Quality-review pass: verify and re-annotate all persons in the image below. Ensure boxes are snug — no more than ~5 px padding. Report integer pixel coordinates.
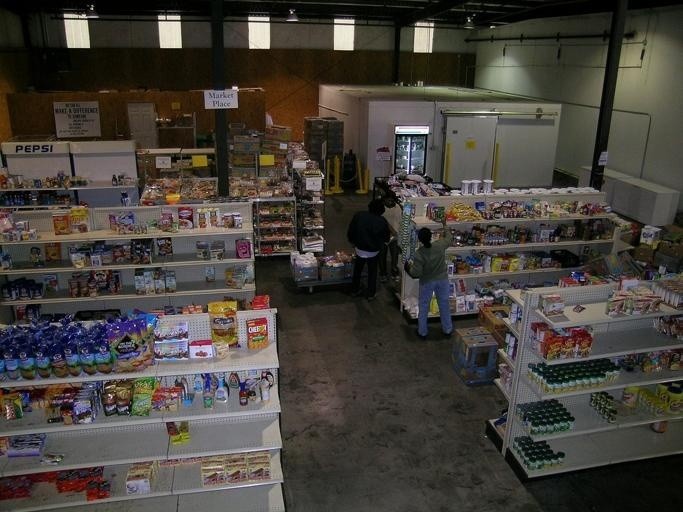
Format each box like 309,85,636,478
408,217,454,339
378,190,403,282
347,198,390,301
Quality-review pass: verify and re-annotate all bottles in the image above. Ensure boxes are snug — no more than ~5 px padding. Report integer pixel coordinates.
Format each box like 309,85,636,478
512,359,621,474
513,225,520,243
203,371,274,410
112,175,117,186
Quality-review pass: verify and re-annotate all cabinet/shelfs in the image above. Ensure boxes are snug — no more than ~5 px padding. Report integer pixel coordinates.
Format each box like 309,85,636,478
578,166,680,227
1,168,220,205
0,307,286,510
484,271,683,478
399,187,622,322
0,200,256,325
228,141,327,255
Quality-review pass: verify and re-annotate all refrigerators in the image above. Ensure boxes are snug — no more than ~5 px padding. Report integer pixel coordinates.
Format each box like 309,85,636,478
395,125,428,175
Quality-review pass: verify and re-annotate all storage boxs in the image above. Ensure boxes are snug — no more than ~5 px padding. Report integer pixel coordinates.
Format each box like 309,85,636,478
231,124,292,150
305,117,344,182
289,262,354,281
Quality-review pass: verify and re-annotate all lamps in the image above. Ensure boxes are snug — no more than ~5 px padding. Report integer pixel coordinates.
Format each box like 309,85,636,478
463,17,475,29
85,5,99,18
286,9,299,22
